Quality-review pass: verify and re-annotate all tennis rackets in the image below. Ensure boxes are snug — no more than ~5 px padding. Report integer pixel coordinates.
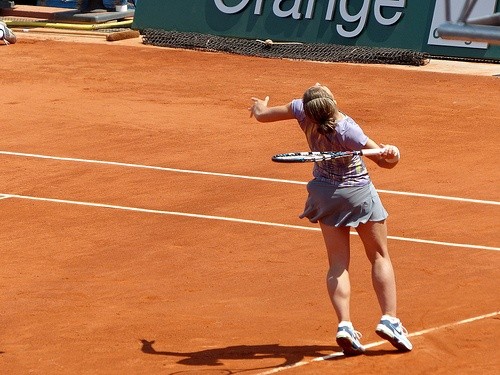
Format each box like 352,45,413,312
271,147,386,164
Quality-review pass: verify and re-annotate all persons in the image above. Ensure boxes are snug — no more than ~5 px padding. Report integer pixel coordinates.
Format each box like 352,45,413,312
248,82,412,353
0,22,17,45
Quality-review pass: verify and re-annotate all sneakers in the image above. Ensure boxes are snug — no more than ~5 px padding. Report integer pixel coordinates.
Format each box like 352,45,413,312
336,327,366,355
375,320,413,351
0,21,17,46
